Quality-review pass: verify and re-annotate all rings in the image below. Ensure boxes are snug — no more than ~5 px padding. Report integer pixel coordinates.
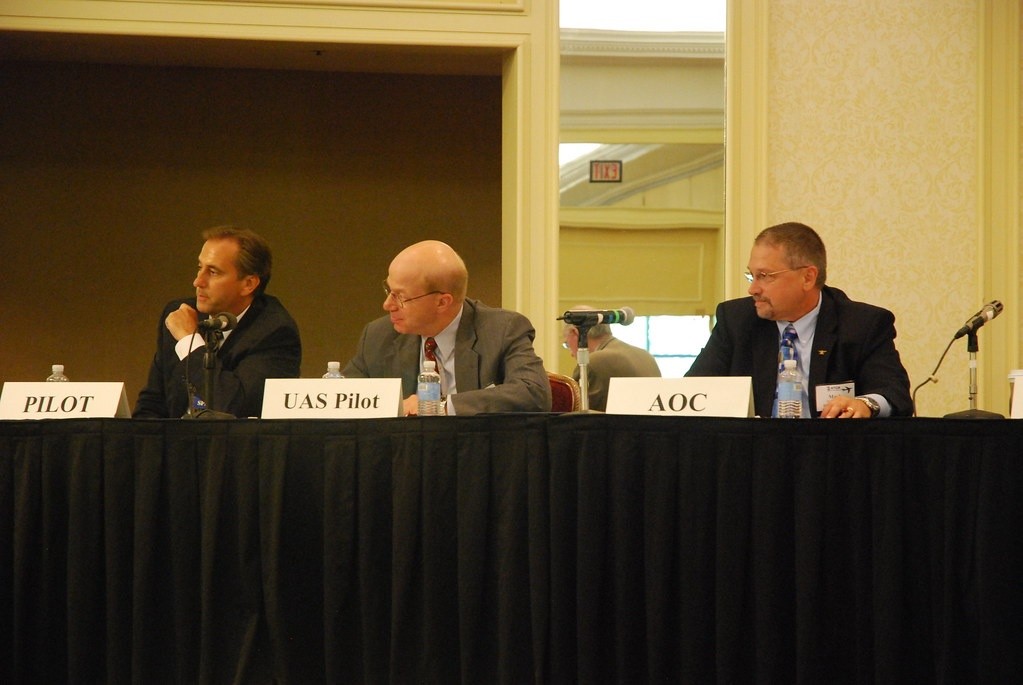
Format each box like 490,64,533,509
847,407,854,412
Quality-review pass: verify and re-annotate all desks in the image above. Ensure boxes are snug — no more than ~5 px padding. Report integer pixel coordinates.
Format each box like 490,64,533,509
0,409,1023,685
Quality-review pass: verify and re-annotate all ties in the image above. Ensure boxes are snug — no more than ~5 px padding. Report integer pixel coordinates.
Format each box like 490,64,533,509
424,337,440,374
779,324,813,418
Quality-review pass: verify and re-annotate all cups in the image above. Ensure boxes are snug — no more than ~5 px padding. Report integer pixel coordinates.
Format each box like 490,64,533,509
1008,368,1023,420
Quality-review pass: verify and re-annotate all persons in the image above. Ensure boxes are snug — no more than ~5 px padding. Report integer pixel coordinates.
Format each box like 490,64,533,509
562,305,661,412
132,225,301,418
341,240,552,416
684,222,915,418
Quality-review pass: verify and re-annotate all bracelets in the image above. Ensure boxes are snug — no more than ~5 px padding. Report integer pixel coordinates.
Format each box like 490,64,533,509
439,396,447,415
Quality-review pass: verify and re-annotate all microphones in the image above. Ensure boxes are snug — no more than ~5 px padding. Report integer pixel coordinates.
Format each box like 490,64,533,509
557,306,635,326
196,312,238,331
955,299,1004,339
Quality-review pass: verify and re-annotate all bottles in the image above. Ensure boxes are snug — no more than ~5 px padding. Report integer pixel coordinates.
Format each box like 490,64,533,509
46,364,70,382
417,361,441,417
779,360,802,419
321,361,344,379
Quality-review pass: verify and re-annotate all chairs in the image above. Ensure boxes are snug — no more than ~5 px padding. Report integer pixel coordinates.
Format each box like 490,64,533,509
546,369,582,411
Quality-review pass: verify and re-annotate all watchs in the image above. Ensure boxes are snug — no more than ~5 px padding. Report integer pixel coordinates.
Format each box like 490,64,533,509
856,397,880,417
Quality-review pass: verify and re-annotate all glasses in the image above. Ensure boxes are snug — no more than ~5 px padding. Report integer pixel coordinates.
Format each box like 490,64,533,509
382,280,445,308
744,265,807,285
563,336,569,349
